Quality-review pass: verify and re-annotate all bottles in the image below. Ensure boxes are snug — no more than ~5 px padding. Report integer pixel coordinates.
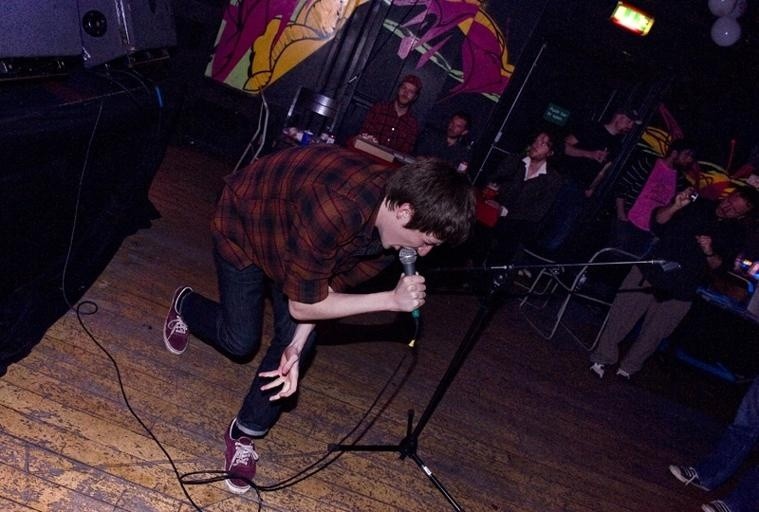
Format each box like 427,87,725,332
455,158,470,173
320,129,337,144
573,270,589,292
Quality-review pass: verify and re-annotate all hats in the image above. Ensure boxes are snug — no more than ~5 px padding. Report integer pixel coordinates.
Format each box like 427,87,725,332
617,106,644,120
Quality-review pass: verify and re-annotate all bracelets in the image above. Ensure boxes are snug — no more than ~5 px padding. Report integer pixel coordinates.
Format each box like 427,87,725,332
703,245,720,258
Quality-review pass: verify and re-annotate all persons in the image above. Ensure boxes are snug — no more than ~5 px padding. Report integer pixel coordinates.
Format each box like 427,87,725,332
542,102,645,260
602,131,701,257
360,72,424,165
665,374,758,511
485,124,565,266
414,110,481,178
587,183,758,382
159,139,479,497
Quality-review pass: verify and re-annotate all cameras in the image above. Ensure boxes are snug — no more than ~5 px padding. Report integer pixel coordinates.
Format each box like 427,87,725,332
690,193,700,203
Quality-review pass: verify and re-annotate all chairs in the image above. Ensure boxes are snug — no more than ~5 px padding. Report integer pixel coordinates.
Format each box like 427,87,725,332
518,246,641,352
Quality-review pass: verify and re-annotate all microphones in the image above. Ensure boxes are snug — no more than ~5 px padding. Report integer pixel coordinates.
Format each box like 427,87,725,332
400,247,419,318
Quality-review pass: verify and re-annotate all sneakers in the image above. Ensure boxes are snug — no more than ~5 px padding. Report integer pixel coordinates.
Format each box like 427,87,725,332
590,361,605,379
616,368,631,380
668,465,710,492
162,284,193,355
702,497,732,512
225,417,259,495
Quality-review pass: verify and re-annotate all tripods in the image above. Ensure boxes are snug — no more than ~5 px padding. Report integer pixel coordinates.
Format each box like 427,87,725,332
328,258,633,512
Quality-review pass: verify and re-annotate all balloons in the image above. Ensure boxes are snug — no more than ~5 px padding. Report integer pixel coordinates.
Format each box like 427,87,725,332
708,0,749,47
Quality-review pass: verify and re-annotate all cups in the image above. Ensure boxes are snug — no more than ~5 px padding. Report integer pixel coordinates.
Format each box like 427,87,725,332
302,130,314,145
483,182,500,200
